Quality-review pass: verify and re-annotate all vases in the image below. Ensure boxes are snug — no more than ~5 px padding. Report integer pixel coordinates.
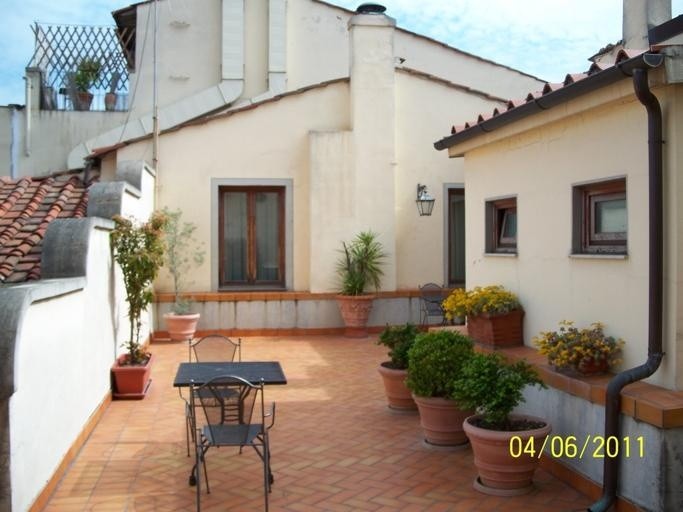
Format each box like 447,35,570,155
465,307,524,347
577,352,609,374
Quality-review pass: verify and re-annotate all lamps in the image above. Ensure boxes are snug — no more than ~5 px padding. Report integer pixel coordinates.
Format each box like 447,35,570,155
415,183,436,216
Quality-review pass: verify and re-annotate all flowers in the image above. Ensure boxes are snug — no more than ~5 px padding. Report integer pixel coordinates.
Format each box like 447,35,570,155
531,320,625,372
440,284,520,321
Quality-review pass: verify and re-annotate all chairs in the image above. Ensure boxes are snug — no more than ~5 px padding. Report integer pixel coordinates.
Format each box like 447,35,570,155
178,334,252,457
418,283,448,330
187,375,275,512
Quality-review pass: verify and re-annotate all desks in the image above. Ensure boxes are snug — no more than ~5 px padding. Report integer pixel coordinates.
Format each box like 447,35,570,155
173,362,287,485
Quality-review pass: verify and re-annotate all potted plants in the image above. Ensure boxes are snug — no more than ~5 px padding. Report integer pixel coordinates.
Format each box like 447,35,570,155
111,210,168,400
373,321,419,413
72,60,101,111
403,327,475,452
104,73,120,111
453,351,552,497
326,228,392,338
162,206,206,342
